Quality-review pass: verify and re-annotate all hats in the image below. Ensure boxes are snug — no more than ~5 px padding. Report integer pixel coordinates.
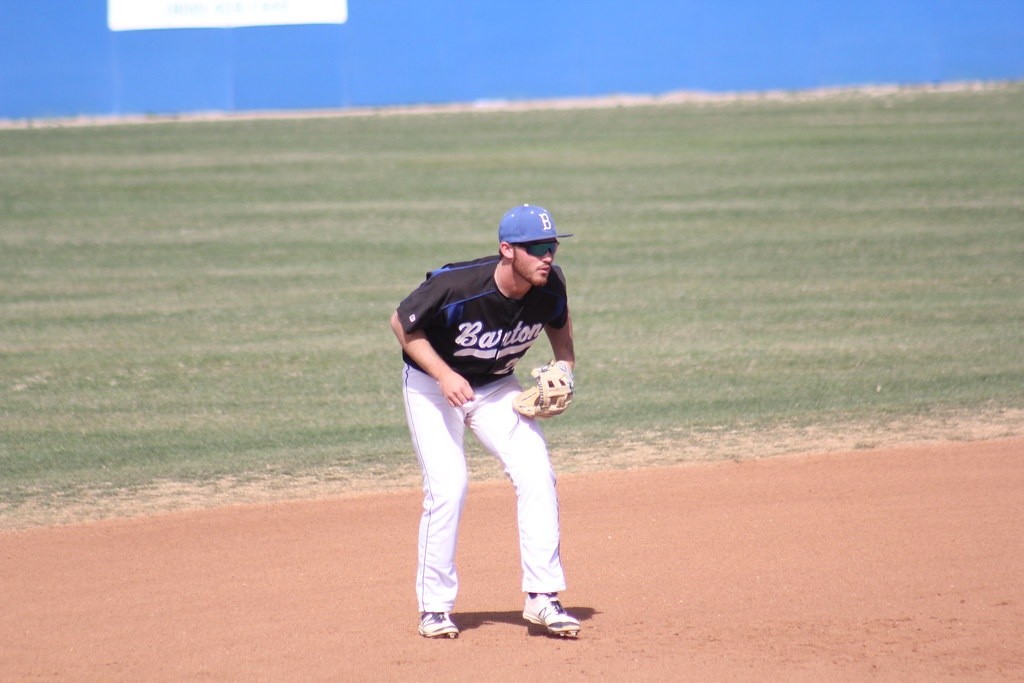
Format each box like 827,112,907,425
498,204,573,244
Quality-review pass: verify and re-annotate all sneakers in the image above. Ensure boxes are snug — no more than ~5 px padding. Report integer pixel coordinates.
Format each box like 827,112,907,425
522,593,581,637
417,611,459,639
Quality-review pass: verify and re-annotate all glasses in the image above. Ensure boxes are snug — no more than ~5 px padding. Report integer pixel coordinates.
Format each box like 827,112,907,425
514,240,559,257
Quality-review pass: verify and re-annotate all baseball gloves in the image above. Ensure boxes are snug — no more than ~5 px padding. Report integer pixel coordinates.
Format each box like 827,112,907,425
512,360,575,420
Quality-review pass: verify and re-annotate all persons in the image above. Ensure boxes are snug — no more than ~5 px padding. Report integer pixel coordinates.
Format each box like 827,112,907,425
390,203,582,640
